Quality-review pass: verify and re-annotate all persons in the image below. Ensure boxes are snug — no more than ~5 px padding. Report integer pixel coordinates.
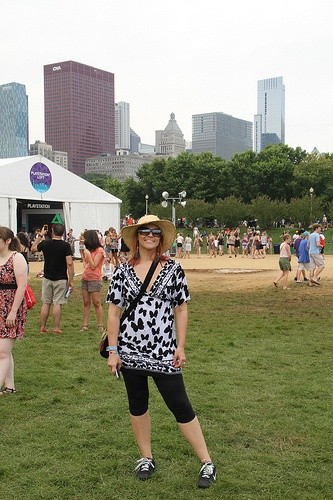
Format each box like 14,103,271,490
105,214,216,487
0,214,329,394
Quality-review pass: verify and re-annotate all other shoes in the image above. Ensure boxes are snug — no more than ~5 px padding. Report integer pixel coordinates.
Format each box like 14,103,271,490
0,387,16,395
98,325,104,332
79,326,88,331
53,329,63,333
310,278,321,285
39,328,48,333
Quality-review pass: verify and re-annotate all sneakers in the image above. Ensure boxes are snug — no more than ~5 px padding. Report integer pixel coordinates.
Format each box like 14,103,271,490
133,456,155,480
197,458,216,488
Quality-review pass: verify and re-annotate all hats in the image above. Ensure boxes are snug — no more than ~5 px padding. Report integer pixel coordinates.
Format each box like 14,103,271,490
121,215,176,253
302,231,309,238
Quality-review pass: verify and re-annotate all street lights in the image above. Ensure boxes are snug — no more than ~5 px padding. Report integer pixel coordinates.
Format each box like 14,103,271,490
161,190,186,228
309,187,314,224
145,195,149,215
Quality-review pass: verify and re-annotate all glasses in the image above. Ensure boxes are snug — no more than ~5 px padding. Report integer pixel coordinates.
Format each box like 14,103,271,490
137,227,162,237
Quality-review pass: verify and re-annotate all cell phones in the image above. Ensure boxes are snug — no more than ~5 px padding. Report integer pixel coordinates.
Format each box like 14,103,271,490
115,366,120,379
44,224,48,234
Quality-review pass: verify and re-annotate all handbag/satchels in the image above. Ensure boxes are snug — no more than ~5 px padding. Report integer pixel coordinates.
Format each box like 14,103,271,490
99,327,113,358
25,285,36,309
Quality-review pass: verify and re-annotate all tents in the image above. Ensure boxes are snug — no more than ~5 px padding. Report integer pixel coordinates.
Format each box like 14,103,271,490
0,155,123,260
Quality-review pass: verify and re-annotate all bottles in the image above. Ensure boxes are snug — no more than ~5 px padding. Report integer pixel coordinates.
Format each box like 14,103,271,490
65,286,73,298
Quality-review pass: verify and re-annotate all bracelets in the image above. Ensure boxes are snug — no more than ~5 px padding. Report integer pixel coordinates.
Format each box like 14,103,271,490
105,346,118,356
38,234,43,238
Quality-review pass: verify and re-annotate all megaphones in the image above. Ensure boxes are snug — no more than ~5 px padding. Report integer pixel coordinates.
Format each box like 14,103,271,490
161,200,168,208
161,191,169,199
178,200,186,208
178,190,187,198
